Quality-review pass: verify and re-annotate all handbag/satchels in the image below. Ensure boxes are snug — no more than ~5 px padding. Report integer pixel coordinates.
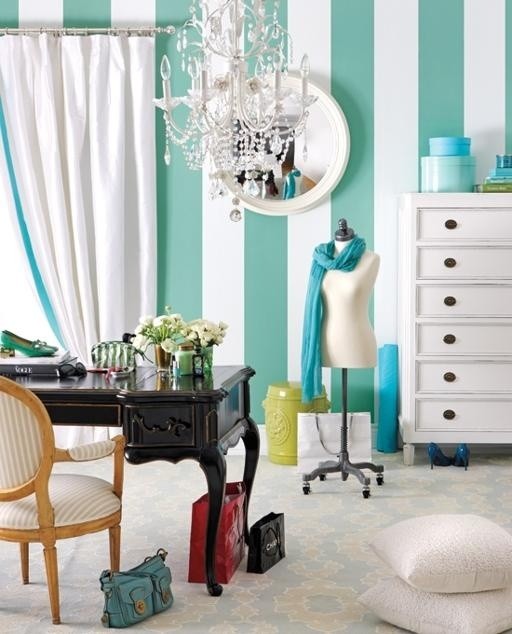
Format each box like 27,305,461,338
100,548,173,628
188,481,246,583
247,512,285,573
92,340,136,369
297,412,372,473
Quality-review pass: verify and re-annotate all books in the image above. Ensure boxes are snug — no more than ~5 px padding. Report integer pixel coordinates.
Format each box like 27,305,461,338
474,177,512,193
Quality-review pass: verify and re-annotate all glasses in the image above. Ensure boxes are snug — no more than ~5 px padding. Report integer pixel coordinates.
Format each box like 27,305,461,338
59,363,87,378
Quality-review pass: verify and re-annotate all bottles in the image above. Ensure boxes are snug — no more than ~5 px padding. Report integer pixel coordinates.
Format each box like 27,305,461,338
192,347,203,379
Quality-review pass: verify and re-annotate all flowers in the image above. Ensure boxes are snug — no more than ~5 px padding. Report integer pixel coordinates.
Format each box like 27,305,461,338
170,316,231,349
129,305,188,354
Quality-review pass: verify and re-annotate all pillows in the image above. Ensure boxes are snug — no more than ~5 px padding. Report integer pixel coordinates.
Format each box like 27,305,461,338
355,575,512,634
363,511,512,596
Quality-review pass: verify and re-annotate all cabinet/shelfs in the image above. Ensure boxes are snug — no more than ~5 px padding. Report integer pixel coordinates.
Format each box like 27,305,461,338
393,190,512,469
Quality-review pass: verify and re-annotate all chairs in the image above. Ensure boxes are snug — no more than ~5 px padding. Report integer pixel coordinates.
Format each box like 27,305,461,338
0,375,129,627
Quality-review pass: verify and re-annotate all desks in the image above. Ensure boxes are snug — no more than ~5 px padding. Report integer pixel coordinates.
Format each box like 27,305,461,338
8,363,262,599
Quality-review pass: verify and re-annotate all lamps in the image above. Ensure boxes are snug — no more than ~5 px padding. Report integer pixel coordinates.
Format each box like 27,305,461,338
158,1,322,225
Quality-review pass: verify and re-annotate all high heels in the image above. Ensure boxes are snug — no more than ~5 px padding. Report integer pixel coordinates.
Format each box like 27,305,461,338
1,330,58,357
427,443,453,469
453,444,470,470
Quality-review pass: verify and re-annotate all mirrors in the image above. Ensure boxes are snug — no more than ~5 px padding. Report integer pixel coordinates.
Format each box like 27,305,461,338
209,71,354,218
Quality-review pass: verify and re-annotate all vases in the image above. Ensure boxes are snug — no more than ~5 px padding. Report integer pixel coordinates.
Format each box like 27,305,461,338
202,346,215,371
153,345,174,373
